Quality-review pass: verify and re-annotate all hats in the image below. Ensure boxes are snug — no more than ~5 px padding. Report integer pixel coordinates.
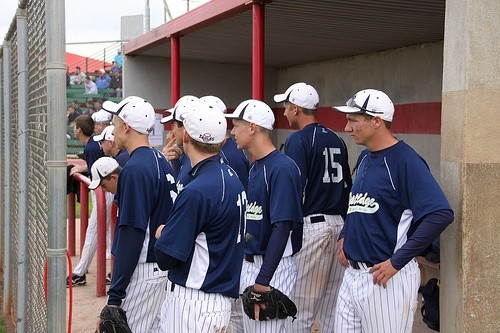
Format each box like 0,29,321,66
166,94,199,113
201,96,227,113
88,157,119,189
94,108,111,123
93,125,115,142
273,82,319,110
180,106,227,144
332,88,395,123
160,104,192,123
224,99,275,131
102,96,155,135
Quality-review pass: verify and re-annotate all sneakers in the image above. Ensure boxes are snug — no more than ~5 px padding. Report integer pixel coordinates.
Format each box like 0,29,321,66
66,273,87,287
104,274,112,284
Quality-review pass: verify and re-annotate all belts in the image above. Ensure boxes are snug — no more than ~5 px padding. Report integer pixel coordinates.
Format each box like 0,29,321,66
311,216,325,223
244,254,265,263
348,259,376,269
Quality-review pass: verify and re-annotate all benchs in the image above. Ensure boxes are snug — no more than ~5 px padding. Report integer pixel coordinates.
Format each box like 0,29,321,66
67,67,122,158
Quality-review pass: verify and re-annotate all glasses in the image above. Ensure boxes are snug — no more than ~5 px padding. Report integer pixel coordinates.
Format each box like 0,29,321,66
346,97,382,114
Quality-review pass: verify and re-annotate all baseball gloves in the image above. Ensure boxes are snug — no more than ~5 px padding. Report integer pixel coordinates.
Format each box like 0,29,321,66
241,285,298,322
95,305,132,333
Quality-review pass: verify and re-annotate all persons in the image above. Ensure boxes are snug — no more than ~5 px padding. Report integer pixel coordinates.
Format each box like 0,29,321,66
223,100,304,333
274,83,352,333
66,96,250,333
66,49,122,99
333,88,454,333
